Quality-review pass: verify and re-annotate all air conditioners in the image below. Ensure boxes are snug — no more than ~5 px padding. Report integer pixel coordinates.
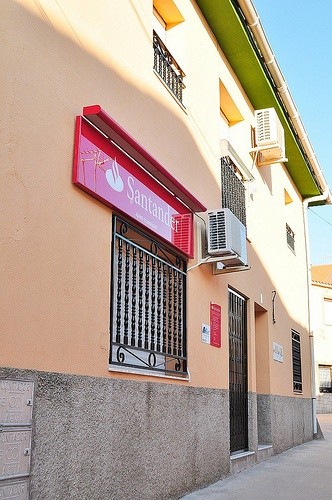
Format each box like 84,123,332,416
254,109,286,162
207,210,250,267
